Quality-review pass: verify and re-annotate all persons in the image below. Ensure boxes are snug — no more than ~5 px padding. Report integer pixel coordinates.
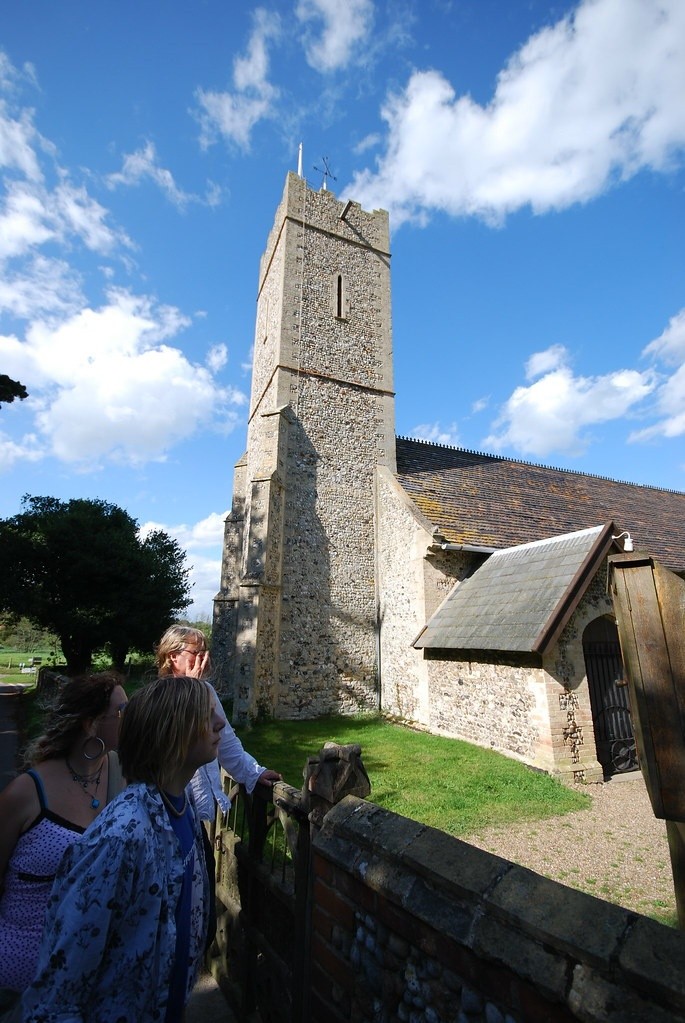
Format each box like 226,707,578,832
23,674,230,1023
145,621,282,952
0,674,130,1006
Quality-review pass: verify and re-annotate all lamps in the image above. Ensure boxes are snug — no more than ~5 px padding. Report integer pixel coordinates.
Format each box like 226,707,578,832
612,530,633,551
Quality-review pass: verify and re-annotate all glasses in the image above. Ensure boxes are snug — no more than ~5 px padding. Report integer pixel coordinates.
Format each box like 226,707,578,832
183,649,205,657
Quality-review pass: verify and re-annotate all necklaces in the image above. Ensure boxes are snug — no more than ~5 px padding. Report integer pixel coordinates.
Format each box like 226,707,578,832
64,754,105,809
156,781,188,816
